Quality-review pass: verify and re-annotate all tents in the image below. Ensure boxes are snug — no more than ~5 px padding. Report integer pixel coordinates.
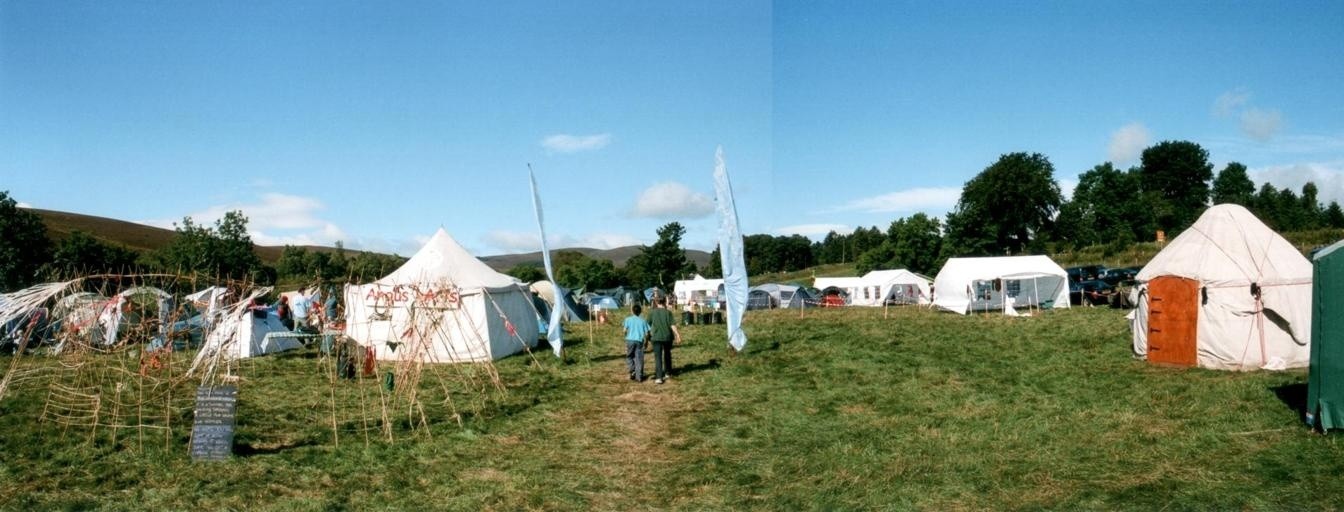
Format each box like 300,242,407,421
340,224,541,365
528,274,860,335
0,284,323,363
849,268,933,307
1126,201,1314,373
1302,237,1344,434
931,253,1071,316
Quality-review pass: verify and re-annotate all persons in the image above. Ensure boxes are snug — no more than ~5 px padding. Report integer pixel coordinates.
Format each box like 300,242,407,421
325,284,342,329
646,293,682,385
621,304,651,382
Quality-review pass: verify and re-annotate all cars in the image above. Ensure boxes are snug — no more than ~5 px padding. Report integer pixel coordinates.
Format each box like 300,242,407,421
1065,263,1143,308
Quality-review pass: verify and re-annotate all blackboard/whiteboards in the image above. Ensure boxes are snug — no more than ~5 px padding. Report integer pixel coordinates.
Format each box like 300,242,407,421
186,386,238,463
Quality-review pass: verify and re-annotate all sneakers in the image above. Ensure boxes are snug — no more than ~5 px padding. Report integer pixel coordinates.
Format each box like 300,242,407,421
624,371,674,385
